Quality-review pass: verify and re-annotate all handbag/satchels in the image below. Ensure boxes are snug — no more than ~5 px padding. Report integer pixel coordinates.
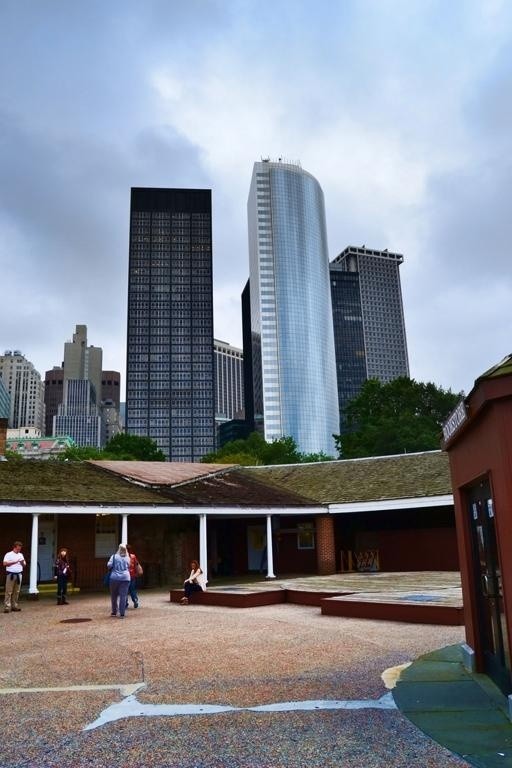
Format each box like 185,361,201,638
134,560,143,577
103,567,113,585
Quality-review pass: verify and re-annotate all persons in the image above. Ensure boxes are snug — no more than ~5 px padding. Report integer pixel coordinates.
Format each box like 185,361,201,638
126,544,139,608
107,543,131,618
54,547,70,605
3,540,26,613
180,560,206,605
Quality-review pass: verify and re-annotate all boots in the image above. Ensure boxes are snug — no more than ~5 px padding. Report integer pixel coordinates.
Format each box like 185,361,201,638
57,596,68,605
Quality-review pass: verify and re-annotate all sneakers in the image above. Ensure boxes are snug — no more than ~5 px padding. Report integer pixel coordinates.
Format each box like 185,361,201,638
180,597,188,604
4,608,21,613
111,601,138,618
